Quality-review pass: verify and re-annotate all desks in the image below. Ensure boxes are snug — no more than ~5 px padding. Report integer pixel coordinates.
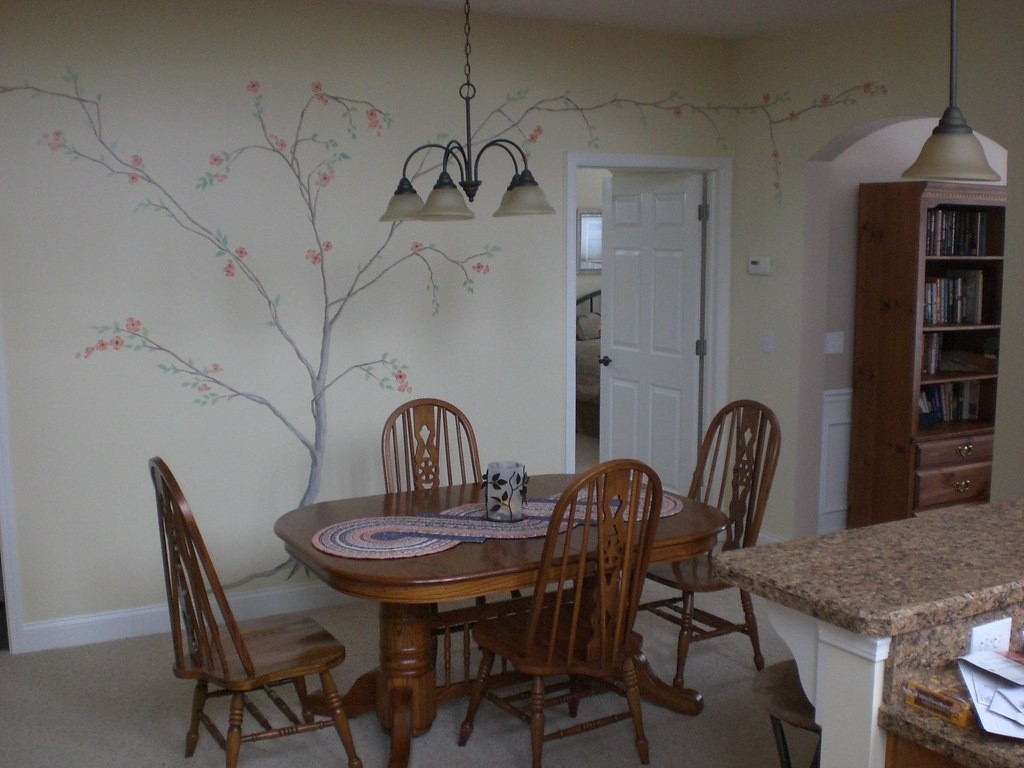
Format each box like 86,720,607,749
274,473,729,768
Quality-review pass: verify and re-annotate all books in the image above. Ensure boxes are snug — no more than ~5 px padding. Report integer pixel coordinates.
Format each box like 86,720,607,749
921,332,944,374
927,209,988,256
920,382,980,423
924,270,983,327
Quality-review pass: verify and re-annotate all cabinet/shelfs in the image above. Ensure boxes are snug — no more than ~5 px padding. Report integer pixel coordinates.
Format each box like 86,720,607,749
844,182,1008,530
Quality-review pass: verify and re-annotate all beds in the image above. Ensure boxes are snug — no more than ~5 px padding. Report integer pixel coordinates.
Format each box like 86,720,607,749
576,290,601,438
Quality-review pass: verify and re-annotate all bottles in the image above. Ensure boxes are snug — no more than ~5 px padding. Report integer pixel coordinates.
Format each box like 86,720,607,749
481,461,530,522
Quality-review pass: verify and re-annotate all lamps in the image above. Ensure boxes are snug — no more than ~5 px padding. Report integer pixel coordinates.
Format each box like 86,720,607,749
378,0,559,221
901,0,1001,181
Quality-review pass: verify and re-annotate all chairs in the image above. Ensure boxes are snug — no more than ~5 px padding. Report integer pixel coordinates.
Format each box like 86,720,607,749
642,399,781,690
381,399,524,653
148,457,364,768
455,458,662,768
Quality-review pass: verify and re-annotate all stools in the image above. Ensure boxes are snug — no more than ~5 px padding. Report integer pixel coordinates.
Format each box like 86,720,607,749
753,658,822,768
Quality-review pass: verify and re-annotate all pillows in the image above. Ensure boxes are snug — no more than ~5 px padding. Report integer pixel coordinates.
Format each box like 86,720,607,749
576,312,601,341
